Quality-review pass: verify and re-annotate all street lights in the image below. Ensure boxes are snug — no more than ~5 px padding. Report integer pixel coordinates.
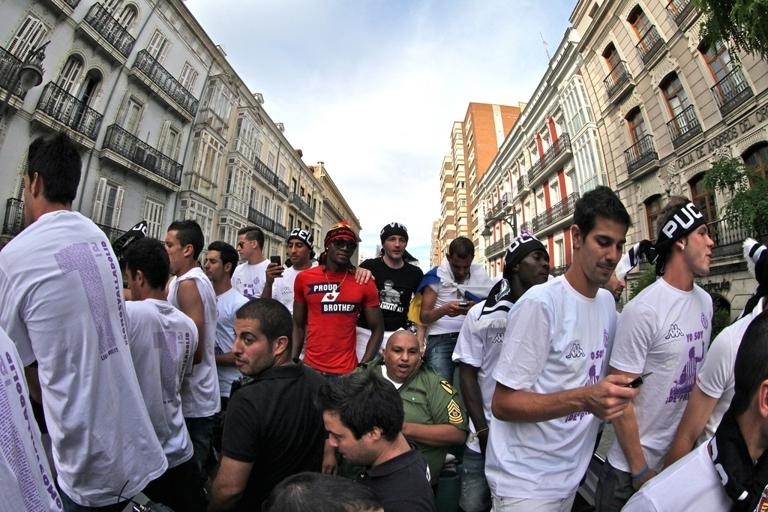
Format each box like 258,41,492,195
1,36,53,122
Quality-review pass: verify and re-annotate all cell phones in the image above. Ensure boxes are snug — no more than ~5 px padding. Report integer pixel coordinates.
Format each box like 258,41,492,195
459,302,468,307
270,256,282,275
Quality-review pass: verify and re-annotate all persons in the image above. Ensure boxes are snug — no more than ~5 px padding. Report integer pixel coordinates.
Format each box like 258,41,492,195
669,341,704,402
663,238,768,469
594,196,714,512
0,132,170,512
585,360,604,385
484,186,640,512
203,299,470,512
0,327,64,512
604,271,627,303
124,236,200,504
123,220,222,465
204,221,556,484
621,309,768,512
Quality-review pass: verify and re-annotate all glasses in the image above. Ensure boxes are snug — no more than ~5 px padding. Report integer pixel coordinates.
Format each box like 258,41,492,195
330,238,357,251
239,239,252,249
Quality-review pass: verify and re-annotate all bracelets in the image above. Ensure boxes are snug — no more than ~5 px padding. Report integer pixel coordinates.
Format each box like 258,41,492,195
631,465,649,480
